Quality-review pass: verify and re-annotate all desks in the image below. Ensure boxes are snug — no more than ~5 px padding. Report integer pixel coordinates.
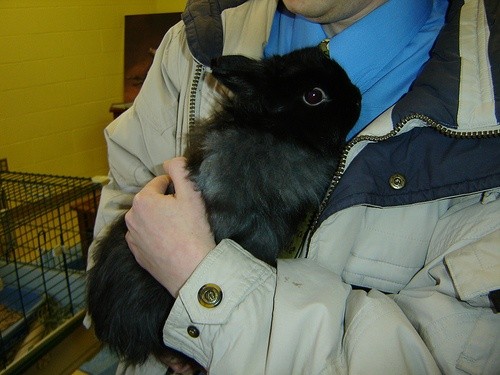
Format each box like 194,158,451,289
110,102,133,120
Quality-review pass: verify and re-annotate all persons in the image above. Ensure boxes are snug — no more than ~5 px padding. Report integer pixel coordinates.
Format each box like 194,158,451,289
83,0,500,374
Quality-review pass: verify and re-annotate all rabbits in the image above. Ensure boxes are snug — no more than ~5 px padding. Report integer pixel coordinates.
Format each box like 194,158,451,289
86,43,362,366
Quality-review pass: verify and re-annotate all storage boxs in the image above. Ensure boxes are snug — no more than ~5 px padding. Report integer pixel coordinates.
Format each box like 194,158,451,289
70,193,100,258
